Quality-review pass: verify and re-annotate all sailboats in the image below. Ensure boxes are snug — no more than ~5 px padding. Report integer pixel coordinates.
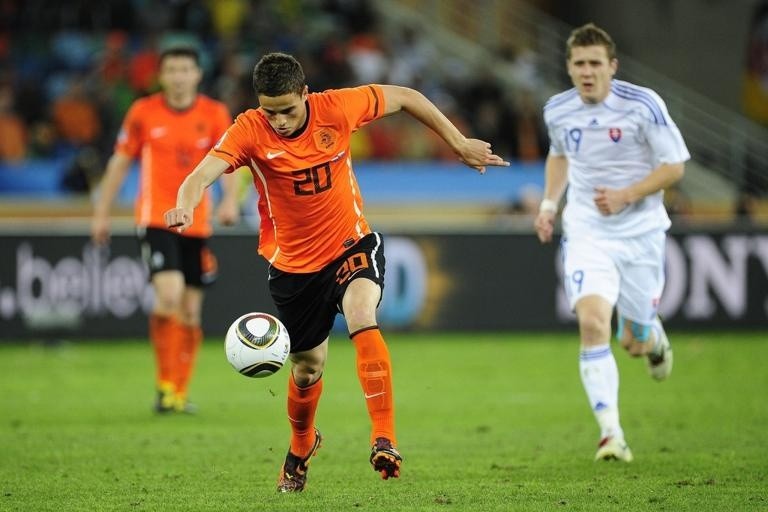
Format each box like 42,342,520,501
225,312,290,378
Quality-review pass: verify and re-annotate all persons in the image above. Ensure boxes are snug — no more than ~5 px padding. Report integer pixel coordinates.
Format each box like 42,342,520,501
162,53,512,496
531,21,692,464
90,42,241,416
0,0,766,226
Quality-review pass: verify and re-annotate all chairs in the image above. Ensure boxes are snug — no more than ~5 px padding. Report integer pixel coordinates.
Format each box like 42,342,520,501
593,432,635,467
275,426,322,494
645,311,672,382
369,434,403,482
155,382,187,413
